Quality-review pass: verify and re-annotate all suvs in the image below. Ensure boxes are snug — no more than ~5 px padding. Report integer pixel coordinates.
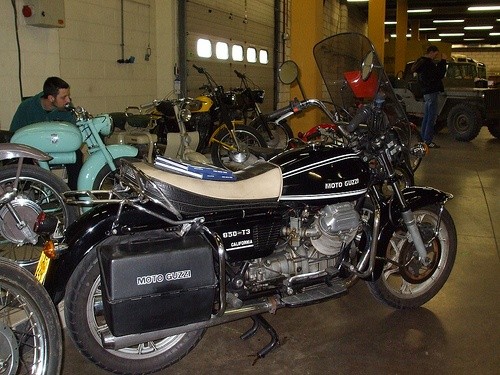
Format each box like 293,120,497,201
391,57,500,142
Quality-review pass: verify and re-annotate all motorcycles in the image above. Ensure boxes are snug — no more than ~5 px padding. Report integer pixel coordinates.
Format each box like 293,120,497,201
9,103,138,214
0,142,81,271
97,96,209,166
150,64,268,172
0,257,65,375
219,70,295,157
62,61,457,375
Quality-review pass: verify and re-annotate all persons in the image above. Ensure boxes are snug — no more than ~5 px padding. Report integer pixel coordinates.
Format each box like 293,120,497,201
7,76,84,191
415,44,448,149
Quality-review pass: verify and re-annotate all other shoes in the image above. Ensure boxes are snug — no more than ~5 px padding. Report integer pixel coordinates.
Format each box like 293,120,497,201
425,141,440,149
